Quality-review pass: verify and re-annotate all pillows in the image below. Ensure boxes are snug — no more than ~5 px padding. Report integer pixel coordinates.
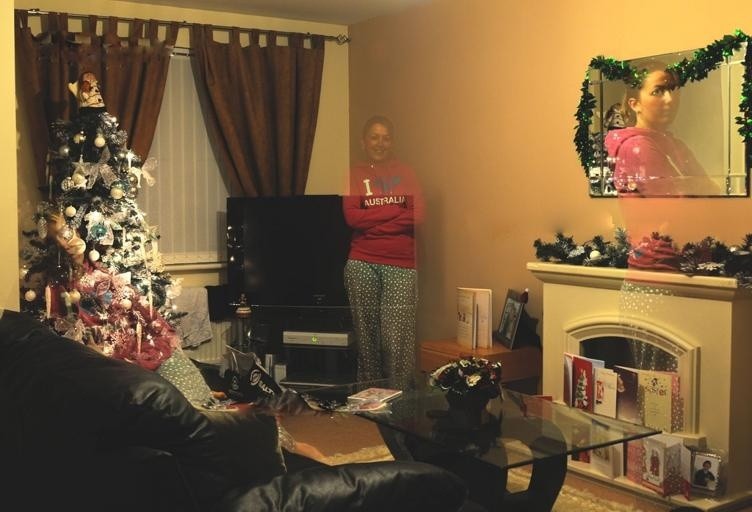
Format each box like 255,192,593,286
155,347,216,412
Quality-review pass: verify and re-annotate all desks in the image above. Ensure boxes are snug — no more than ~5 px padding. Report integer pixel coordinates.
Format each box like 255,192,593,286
419,334,542,396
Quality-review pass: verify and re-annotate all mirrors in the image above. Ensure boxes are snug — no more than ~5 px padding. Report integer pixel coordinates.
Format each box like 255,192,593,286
588,41,751,199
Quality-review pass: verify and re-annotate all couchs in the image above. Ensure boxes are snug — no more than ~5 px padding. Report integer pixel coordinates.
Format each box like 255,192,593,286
0,310,469,511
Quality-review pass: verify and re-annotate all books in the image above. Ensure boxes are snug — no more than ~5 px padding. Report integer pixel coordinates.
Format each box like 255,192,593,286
455,286,494,350
347,386,404,404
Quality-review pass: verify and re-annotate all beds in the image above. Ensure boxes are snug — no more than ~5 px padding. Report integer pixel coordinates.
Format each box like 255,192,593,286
301,369,662,512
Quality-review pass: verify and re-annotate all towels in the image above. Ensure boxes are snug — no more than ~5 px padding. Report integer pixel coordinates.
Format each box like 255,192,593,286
172,287,212,348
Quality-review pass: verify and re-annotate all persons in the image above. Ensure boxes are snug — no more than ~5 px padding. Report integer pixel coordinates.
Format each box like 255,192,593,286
693,460,716,489
500,306,518,339
41,210,255,422
603,61,722,498
342,114,428,422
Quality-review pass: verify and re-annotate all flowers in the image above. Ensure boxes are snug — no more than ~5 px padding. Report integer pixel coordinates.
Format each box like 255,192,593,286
427,355,502,395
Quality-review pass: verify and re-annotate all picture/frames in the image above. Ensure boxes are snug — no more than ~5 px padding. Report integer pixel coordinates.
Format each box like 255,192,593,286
495,289,525,350
691,451,724,492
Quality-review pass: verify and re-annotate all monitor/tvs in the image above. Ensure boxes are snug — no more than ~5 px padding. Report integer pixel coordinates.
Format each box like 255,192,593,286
225,192,420,328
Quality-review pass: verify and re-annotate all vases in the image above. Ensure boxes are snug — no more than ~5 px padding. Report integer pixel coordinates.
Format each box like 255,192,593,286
429,391,503,411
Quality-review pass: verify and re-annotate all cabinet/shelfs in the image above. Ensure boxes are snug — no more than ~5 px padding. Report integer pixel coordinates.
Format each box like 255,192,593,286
231,304,412,402
